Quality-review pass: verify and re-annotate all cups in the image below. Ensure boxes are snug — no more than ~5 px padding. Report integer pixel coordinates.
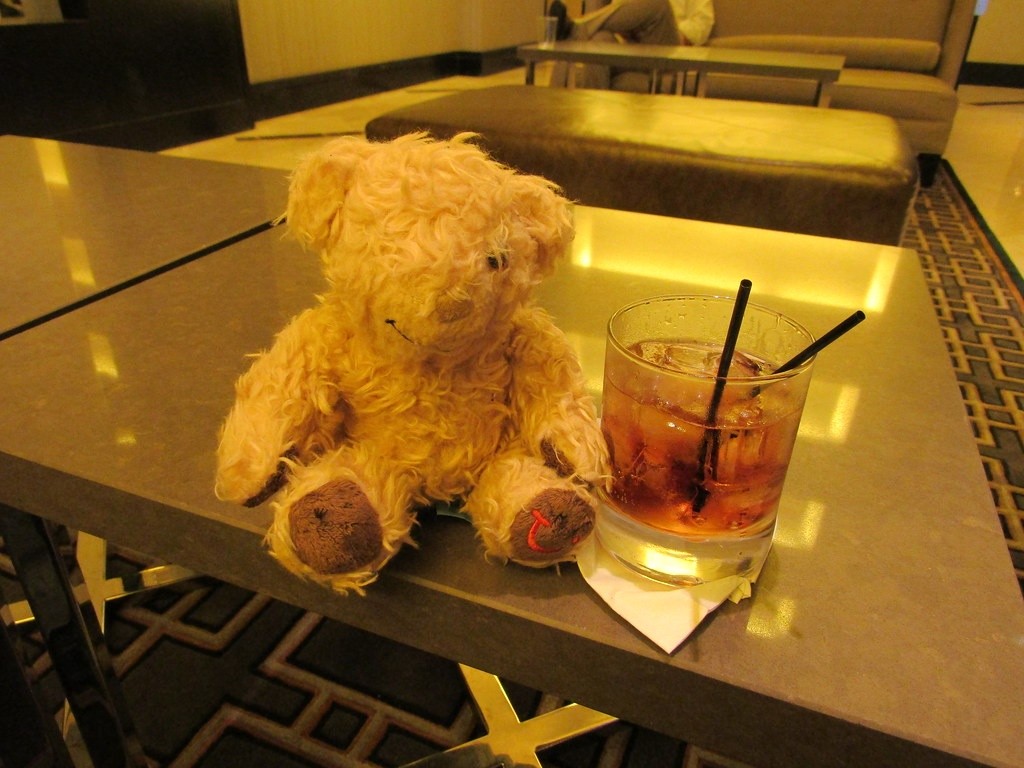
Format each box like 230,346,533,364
596,296,815,586
537,16,558,50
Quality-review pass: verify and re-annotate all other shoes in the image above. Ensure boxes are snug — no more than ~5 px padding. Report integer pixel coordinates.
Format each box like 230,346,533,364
550,0,573,42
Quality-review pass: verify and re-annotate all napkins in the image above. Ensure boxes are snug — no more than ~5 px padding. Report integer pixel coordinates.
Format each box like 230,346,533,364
575,530,771,659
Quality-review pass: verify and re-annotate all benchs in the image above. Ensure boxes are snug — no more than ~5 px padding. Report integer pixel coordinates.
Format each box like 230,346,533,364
544,0,978,188
362,84,920,247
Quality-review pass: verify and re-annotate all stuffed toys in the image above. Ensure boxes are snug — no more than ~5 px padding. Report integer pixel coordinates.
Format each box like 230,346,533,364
214,129,615,596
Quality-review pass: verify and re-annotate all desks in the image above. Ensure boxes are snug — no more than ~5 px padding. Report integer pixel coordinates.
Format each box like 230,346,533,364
519,38,847,109
0,132,1024,768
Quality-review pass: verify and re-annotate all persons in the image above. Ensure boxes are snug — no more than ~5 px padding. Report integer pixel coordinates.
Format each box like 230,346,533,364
542,0,715,94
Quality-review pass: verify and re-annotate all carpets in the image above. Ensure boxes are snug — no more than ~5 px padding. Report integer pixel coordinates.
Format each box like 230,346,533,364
0,159,1024,768
